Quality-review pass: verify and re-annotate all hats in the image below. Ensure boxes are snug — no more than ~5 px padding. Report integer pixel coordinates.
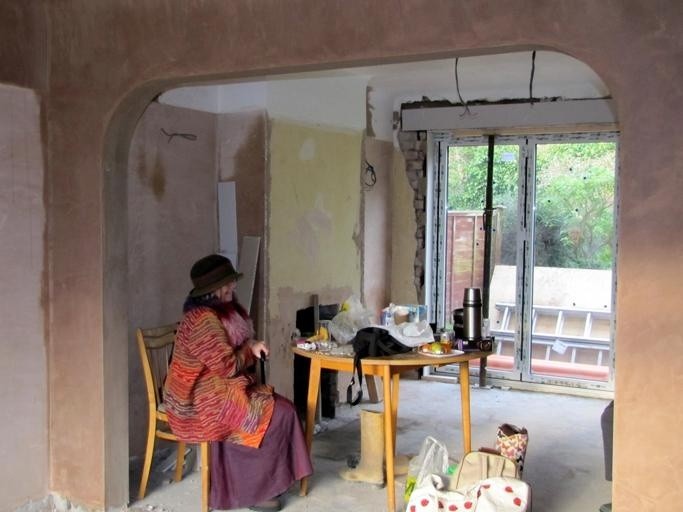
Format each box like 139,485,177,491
187,253,244,296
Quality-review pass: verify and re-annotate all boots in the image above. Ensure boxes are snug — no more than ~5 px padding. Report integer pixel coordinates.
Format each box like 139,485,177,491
337,408,411,490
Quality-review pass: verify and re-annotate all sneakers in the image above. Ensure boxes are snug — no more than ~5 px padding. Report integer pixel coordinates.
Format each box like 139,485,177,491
250,498,281,512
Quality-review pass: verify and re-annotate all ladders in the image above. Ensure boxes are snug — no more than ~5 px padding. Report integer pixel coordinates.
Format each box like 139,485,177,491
491,302,611,368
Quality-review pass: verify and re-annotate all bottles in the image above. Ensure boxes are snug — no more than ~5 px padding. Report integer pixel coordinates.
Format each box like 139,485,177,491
462,287,481,348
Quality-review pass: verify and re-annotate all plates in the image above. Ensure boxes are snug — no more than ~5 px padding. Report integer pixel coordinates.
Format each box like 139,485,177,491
412,347,465,358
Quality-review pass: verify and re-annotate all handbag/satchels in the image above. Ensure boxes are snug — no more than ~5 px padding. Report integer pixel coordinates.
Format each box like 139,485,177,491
450,450,519,493
346,326,413,406
495,423,527,480
329,309,371,343
404,474,530,512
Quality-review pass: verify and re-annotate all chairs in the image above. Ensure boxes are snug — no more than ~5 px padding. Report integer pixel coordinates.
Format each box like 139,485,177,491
136,324,214,511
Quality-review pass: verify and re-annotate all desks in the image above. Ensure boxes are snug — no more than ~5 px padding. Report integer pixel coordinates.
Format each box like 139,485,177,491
288,339,495,511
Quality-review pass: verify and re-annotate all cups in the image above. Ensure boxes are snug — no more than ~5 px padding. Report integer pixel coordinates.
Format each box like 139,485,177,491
314,319,332,353
440,334,452,353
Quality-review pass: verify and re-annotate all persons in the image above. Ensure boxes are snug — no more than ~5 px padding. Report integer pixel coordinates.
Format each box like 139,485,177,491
163,253,314,512
